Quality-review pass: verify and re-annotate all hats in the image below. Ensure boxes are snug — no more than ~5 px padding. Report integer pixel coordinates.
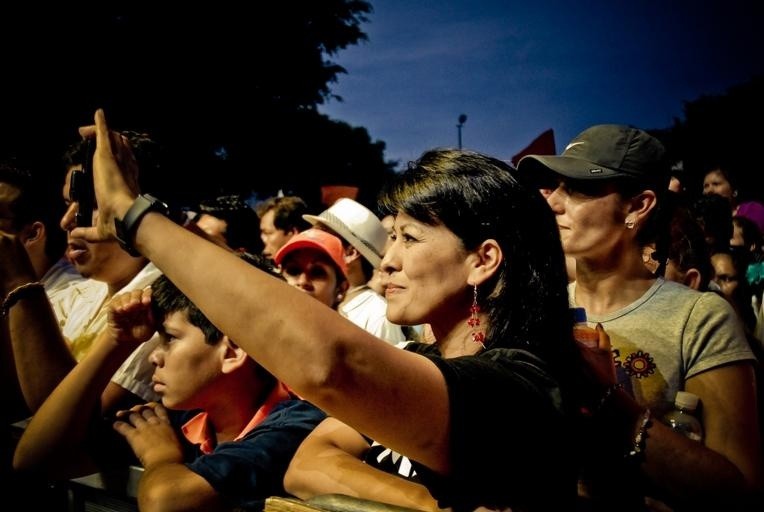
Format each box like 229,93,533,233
732,201,764,234
274,197,388,279
517,124,672,180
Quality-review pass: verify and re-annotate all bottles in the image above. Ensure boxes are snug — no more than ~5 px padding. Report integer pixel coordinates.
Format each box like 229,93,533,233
659,389,705,446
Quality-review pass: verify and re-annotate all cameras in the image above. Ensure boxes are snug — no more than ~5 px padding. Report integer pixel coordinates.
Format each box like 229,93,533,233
67,137,96,227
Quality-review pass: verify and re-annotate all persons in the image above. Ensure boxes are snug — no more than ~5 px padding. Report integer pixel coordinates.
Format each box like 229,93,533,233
1,107,763,511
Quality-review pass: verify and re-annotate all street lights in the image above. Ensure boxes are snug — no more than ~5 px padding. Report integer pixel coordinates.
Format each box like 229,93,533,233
456,114,468,149
